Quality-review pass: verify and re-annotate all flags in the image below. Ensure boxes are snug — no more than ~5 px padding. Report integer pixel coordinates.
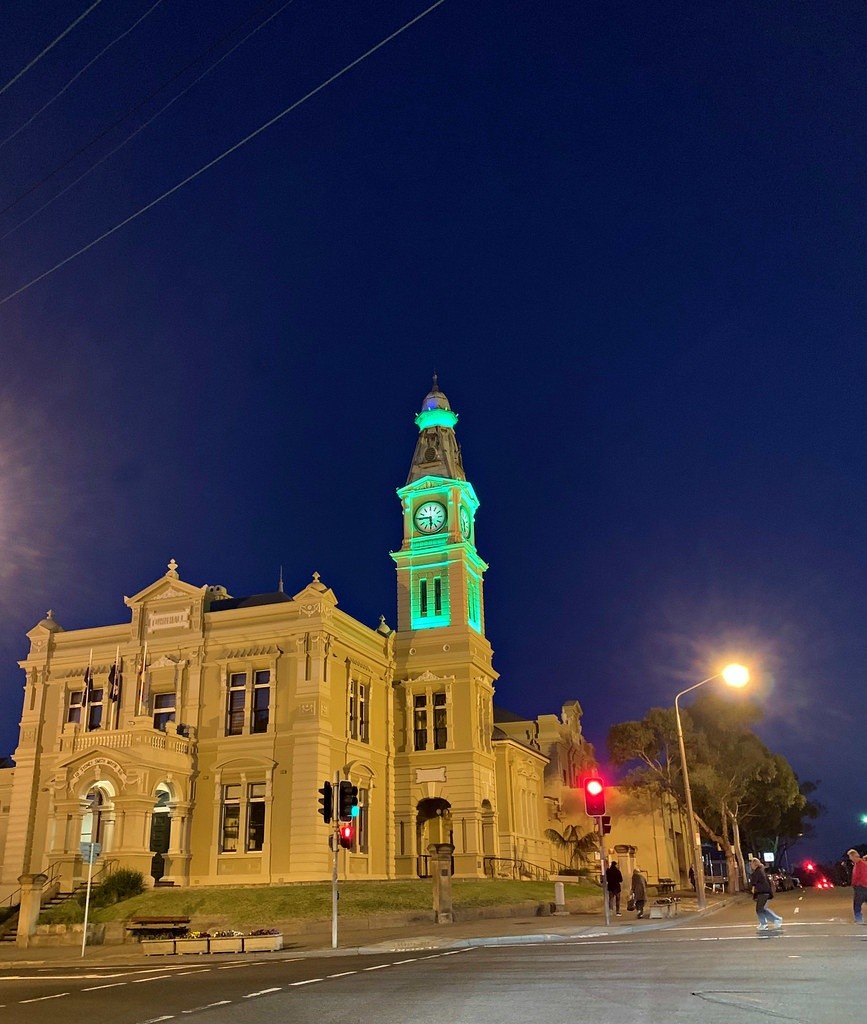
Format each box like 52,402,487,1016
108,654,119,703
138,658,149,702
82,664,94,707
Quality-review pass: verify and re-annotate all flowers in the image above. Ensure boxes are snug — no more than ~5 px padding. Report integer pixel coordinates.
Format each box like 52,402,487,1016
248,928,279,936
177,931,207,939
214,930,244,937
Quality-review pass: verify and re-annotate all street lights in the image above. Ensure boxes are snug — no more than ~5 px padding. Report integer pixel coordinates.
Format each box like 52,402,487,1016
675,663,751,908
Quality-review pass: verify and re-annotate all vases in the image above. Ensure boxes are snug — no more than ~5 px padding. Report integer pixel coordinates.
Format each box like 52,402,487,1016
242,933,283,954
175,937,209,955
209,936,243,954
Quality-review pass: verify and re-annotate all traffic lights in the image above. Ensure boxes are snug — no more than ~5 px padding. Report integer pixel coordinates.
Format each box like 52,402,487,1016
584,776,606,817
600,816,611,836
341,827,353,849
318,781,332,823
339,780,359,823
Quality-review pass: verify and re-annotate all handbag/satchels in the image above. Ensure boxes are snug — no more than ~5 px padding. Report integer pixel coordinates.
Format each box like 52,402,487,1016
627,893,636,911
753,882,774,900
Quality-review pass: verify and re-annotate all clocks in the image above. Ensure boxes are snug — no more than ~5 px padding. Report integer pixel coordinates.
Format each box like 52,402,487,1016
460,505,470,539
413,500,448,535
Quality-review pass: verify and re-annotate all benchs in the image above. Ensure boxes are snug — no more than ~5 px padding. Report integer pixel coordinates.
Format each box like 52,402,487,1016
125,916,188,936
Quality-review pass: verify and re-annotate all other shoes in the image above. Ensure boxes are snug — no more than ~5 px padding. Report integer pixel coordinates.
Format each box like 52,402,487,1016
774,918,782,928
637,909,641,916
616,912,622,916
852,919,865,924
638,915,642,919
756,923,769,930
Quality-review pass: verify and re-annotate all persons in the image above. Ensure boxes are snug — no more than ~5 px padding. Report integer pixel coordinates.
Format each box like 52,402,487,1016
839,849,867,923
605,861,623,916
630,868,647,919
747,858,782,930
689,863,696,892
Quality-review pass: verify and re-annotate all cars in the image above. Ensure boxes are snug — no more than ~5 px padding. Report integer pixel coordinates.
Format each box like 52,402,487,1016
759,866,794,894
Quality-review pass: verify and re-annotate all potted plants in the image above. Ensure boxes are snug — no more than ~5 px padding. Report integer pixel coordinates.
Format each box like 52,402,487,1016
139,934,175,956
